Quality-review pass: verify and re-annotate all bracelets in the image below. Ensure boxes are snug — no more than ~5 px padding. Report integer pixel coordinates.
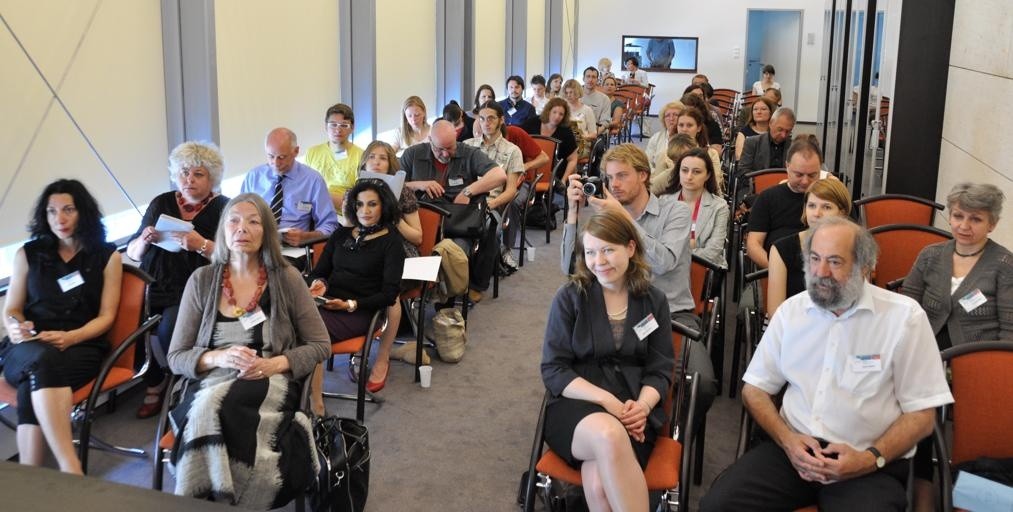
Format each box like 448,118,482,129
199,239,208,254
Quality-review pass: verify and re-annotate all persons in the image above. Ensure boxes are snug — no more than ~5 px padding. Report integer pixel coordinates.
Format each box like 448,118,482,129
646,63,823,269
168,193,332,512
305,178,404,417
561,143,719,444
540,210,676,512
127,141,234,416
247,128,340,274
751,185,848,446
299,58,647,304
899,182,1013,482
646,39,675,69
0,178,121,475
358,143,421,393
696,214,956,512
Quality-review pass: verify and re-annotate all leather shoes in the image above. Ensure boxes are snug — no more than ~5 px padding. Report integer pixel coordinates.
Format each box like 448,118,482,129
136,386,166,418
366,363,390,391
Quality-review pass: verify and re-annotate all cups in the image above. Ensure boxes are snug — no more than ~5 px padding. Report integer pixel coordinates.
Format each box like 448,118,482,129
418,366,433,387
527,247,535,261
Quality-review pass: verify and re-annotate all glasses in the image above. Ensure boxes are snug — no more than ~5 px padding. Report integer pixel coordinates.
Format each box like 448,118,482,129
327,123,351,130
355,178,385,188
429,140,458,153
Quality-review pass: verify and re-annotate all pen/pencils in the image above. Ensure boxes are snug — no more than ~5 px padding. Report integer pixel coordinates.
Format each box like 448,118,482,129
144,233,152,239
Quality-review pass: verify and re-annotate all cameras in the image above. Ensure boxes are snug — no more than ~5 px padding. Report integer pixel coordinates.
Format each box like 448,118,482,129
578,175,603,197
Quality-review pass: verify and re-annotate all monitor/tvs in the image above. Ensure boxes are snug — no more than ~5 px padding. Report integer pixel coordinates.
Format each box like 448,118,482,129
621,35,698,74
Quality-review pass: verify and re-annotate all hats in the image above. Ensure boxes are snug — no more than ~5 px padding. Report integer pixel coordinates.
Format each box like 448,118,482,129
391,341,430,365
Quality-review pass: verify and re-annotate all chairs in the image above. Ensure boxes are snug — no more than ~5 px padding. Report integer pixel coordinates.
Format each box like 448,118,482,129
0,264,155,476
741,307,936,512
155,255,310,493
312,77,657,411
931,339,1012,511
515,319,707,512
677,75,1009,338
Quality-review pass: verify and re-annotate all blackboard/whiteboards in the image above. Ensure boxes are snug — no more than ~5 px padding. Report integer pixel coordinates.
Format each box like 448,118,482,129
9,316,37,335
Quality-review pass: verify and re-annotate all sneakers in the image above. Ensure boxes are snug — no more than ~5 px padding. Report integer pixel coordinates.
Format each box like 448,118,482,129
467,289,483,303
435,297,456,312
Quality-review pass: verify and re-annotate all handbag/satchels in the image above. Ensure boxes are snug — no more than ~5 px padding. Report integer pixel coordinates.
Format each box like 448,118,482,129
493,243,520,277
307,413,371,512
433,307,467,363
418,185,491,239
516,471,587,512
522,200,559,230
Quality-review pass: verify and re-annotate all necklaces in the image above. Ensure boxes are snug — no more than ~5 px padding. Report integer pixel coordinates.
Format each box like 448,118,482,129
222,264,267,318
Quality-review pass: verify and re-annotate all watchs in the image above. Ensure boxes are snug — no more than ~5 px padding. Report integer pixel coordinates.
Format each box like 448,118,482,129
865,447,885,469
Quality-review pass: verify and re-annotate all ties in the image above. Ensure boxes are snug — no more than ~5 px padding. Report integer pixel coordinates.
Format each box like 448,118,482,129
269,175,286,223
